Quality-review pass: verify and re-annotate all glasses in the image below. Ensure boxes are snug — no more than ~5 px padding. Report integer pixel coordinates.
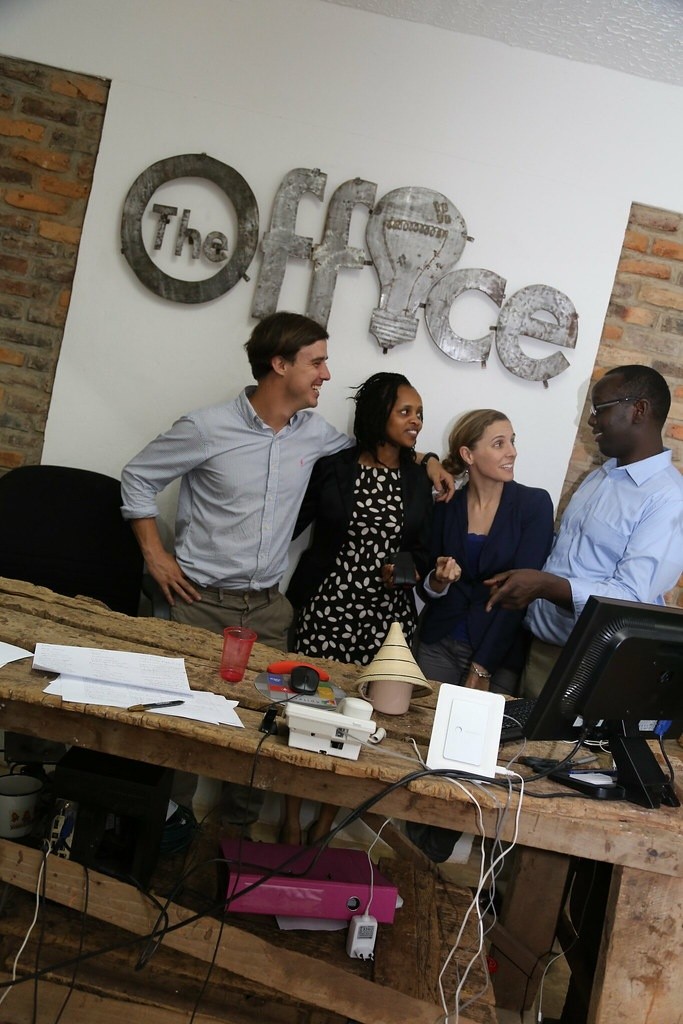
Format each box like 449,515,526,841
590,394,643,417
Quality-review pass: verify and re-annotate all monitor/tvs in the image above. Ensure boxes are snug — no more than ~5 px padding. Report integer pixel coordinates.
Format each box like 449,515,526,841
523,595,683,810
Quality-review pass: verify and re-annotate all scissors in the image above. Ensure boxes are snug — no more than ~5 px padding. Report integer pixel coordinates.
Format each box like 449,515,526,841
518,754,600,774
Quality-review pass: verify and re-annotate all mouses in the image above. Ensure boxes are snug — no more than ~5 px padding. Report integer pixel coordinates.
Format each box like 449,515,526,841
290,666,320,695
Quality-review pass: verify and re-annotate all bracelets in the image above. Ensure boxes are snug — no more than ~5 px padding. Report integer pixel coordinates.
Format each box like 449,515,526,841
470,664,491,680
432,568,448,586
421,452,439,471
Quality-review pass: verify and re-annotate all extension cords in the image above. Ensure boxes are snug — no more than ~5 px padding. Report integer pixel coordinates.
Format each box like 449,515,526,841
49,815,75,861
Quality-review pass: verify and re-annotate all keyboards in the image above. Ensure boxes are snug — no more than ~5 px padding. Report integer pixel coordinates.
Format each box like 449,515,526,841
498,696,540,741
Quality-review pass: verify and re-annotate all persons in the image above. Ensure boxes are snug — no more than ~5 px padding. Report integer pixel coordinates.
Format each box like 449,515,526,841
405,410,554,869
284,373,433,847
119,310,454,859
468,366,683,915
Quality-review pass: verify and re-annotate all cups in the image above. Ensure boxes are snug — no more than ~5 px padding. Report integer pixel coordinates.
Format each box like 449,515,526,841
220,626,257,683
358,680,413,715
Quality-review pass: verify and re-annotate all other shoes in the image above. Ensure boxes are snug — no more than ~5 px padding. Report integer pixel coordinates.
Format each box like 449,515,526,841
481,893,501,916
278,821,302,846
307,820,330,848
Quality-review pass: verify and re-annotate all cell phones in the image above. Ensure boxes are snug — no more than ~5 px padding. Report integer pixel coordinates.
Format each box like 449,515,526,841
393,552,417,586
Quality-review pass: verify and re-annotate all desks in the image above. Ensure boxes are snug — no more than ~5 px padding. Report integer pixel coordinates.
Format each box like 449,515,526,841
0,578,683,1024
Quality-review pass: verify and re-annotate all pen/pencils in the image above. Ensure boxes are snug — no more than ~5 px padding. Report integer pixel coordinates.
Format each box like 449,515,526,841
127,700,185,712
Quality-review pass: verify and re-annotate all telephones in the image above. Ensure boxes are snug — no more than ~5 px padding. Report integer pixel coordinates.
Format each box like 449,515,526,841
281,697,377,762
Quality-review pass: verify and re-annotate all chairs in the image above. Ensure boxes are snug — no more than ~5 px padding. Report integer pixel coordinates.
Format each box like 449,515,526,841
0,464,146,796
